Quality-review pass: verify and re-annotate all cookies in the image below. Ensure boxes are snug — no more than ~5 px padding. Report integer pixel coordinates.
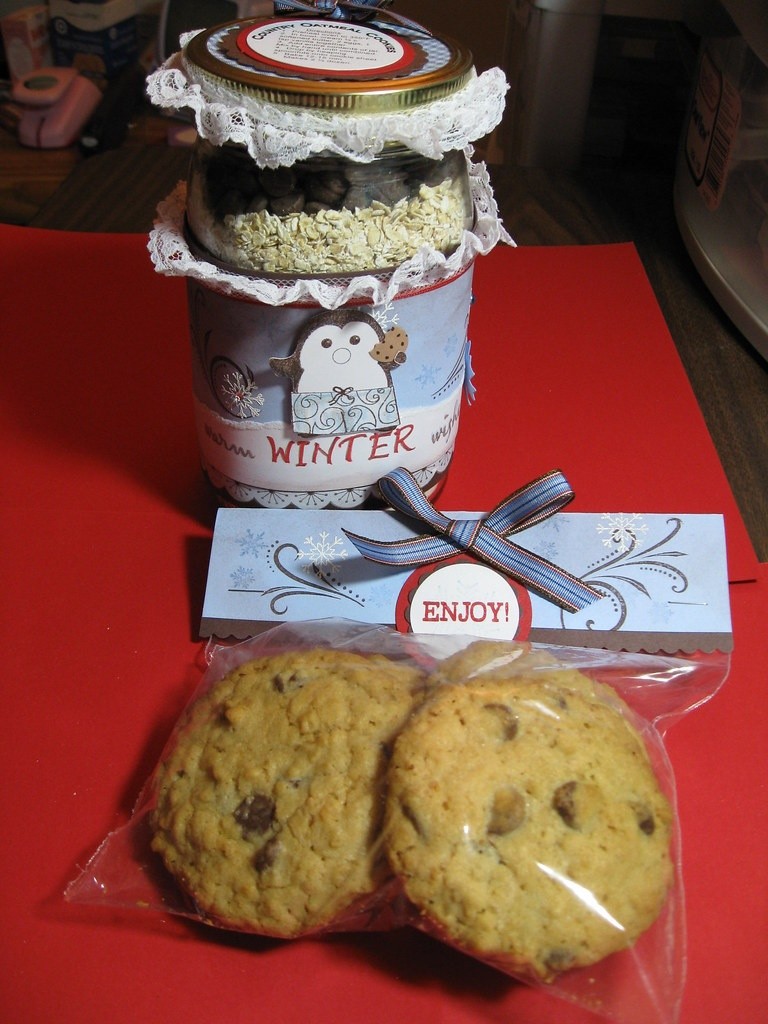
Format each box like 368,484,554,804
149,641,675,979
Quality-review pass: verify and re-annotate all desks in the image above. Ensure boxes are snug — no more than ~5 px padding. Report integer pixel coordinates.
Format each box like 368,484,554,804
0,141,767,1023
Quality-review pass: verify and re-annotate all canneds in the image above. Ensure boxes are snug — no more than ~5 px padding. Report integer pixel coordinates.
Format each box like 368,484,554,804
177,15,477,509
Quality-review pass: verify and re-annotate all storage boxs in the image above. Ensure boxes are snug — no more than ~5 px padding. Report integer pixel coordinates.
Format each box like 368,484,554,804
0,0,167,87
196,506,735,656
